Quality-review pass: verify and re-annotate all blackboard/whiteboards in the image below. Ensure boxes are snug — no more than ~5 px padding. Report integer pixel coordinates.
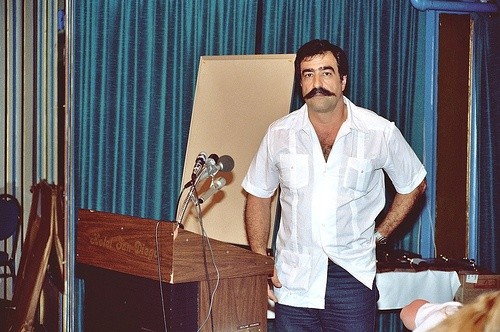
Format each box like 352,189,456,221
174,50,297,248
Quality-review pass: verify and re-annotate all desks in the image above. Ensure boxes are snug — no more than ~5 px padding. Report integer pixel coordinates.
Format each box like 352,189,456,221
376,271,462,332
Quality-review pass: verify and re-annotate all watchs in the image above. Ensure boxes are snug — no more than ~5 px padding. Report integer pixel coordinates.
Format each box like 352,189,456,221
373,230,389,246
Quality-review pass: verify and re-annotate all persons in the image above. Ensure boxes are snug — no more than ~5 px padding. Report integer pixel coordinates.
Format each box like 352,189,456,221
399,288,499,332
240,39,428,332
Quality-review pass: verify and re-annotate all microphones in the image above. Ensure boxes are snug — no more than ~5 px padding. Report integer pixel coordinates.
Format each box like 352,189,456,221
185,151,235,205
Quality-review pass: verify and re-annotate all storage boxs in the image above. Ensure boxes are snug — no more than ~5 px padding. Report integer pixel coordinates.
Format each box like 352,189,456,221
456,274,500,304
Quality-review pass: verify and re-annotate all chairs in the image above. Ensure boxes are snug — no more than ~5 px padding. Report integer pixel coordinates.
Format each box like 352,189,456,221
0,193,21,308
